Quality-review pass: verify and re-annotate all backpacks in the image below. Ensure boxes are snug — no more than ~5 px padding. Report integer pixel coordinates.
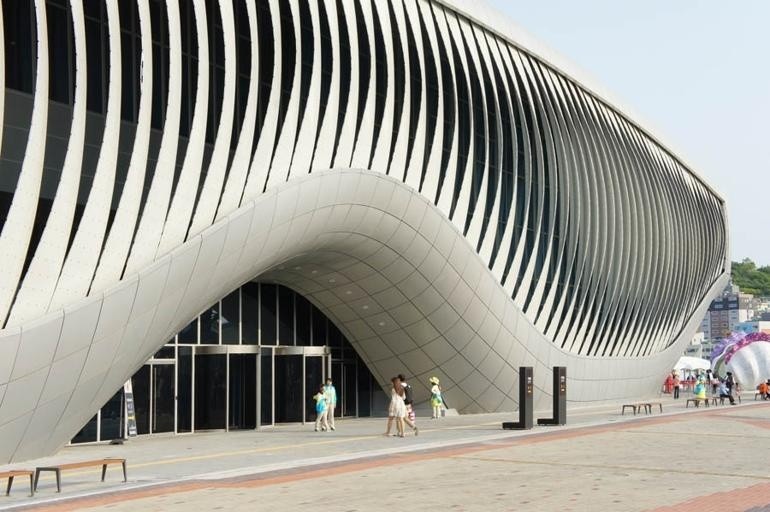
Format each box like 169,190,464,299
401,382,414,404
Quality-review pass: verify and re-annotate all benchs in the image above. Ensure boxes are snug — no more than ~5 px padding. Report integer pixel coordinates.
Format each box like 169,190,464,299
0,470,35,496
34,459,127,493
686,398,709,407
712,396,741,407
622,402,663,416
756,393,764,401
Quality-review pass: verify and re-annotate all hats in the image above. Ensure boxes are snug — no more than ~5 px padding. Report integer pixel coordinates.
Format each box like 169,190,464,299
430,376,439,384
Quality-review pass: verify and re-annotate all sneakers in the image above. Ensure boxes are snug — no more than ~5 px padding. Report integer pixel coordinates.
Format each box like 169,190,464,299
315,426,335,431
385,430,405,437
414,425,418,436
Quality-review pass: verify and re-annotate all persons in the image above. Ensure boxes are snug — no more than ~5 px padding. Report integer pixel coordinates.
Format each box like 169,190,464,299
384,376,409,438
391,374,419,437
429,376,443,419
311,384,332,432
321,377,339,431
662,366,770,407
